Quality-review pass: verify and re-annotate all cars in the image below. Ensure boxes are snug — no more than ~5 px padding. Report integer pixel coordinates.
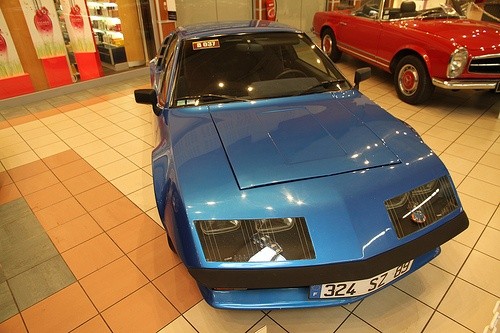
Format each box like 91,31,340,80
312,0,500,104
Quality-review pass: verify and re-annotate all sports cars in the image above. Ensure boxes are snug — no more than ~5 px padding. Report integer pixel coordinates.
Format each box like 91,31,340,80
134,17,471,311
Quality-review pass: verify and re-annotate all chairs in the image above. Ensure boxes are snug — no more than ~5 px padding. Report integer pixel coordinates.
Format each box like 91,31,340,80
178,54,232,96
252,51,296,83
394,1,420,18
